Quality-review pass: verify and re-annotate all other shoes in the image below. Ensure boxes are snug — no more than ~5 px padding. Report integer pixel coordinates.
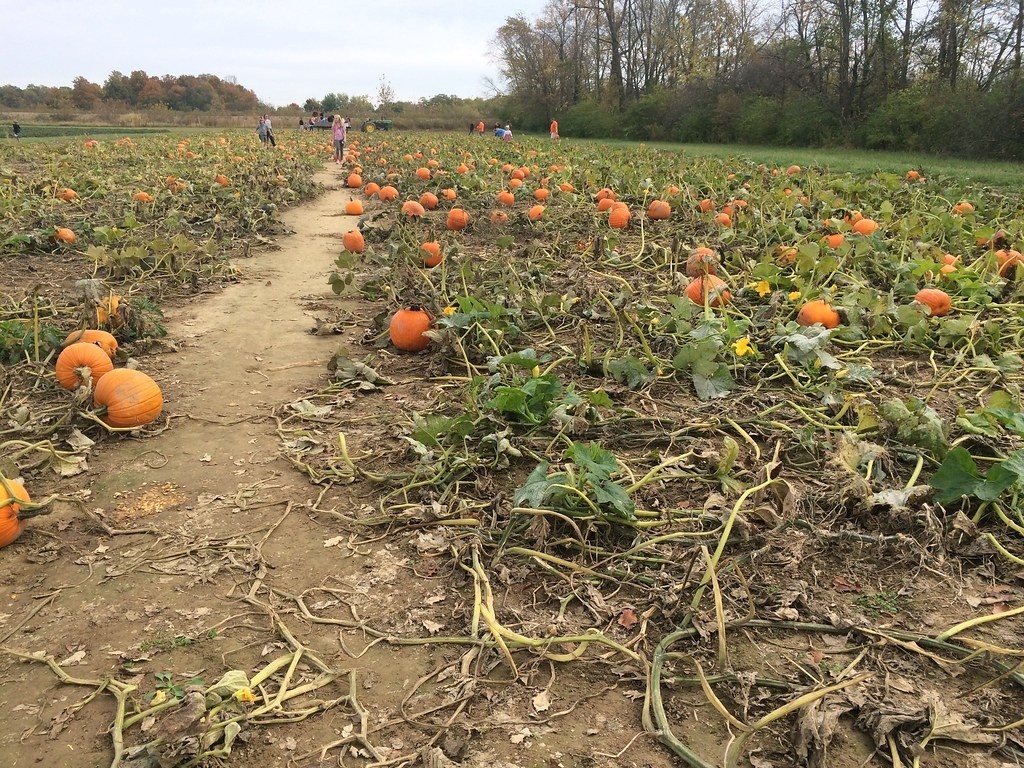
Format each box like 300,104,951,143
340,159,343,164
336,159,340,164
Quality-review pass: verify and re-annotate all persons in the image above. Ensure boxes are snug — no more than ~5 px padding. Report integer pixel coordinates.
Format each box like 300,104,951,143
493,122,506,139
304,121,308,130
319,113,350,123
299,117,304,130
549,117,559,139
263,114,278,149
309,118,314,131
254,116,273,149
12,119,22,142
331,114,347,165
468,122,474,135
478,120,485,137
502,125,513,141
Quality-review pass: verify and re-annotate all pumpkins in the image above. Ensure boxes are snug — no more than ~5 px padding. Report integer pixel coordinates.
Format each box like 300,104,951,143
55,294,162,429
0,477,32,548
50,137,335,245
341,134,1024,351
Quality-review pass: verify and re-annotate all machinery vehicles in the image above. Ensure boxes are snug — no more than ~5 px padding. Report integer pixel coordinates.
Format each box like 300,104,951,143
361,115,392,133
